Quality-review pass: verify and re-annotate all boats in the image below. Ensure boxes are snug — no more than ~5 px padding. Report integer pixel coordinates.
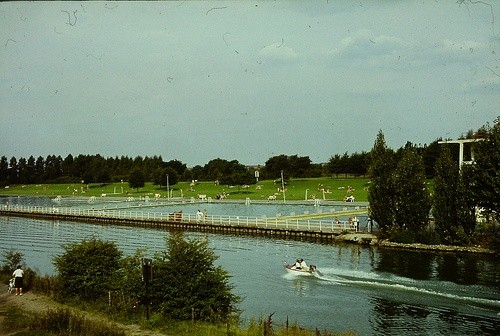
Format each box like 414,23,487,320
51,196,64,202
284,264,316,276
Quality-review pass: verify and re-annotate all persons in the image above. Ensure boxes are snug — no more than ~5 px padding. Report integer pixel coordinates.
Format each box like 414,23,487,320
347,216,360,231
344,184,352,202
196,209,208,224
335,216,342,231
216,191,227,201
291,259,307,269
11,265,25,296
272,193,276,201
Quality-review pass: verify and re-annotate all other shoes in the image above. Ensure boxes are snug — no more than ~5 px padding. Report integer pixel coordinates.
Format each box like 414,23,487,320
20,293,23,295
16,293,18,296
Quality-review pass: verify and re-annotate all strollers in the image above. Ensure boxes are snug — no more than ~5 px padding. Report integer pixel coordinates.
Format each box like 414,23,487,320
8,277,16,293
